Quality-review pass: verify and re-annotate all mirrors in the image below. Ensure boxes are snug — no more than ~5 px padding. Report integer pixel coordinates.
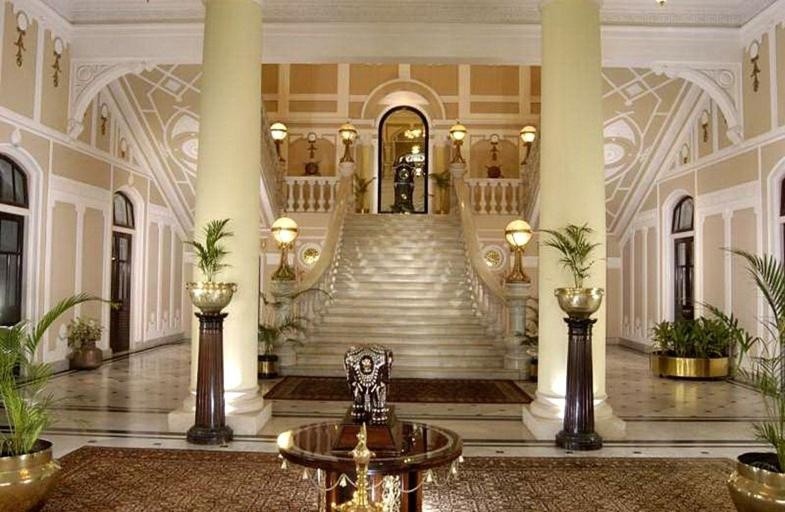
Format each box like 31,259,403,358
374,104,432,215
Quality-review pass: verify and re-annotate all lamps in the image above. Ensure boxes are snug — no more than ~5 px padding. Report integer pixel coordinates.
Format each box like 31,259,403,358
520,123,537,165
403,129,421,139
339,120,359,162
505,219,534,283
448,121,467,163
269,217,300,281
270,122,288,161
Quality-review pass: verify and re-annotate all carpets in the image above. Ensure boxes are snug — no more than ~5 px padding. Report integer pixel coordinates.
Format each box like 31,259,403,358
263,374,534,403
35,444,741,512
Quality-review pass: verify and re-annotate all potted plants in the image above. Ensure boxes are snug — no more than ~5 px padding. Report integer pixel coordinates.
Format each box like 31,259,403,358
537,222,605,321
647,315,744,382
0,291,124,512
65,316,103,370
694,246,785,512
182,219,238,315
258,316,311,379
